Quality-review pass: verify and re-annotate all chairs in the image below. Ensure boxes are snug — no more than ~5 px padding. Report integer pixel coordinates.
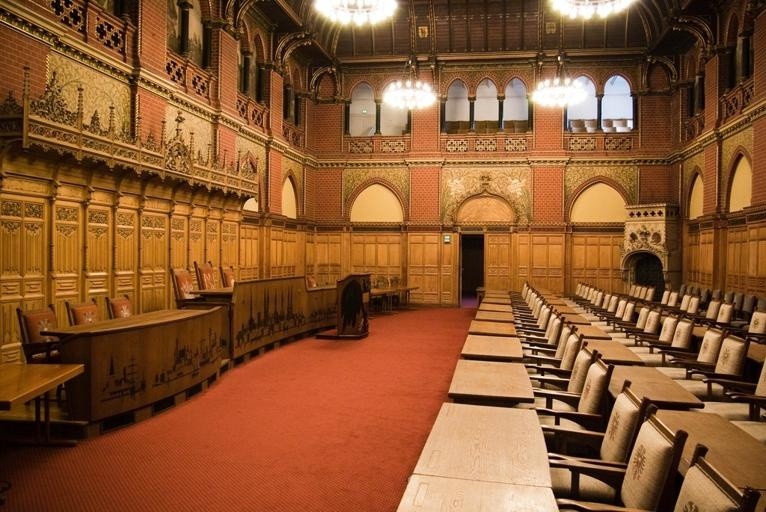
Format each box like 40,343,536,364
14,260,418,406
394,278,765,512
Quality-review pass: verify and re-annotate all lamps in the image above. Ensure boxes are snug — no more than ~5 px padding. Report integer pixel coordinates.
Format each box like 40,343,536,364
382,51,435,114
523,47,586,109
544,0,637,21
310,0,396,27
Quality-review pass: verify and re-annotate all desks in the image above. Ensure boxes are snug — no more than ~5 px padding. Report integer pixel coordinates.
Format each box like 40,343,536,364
0,361,83,497
